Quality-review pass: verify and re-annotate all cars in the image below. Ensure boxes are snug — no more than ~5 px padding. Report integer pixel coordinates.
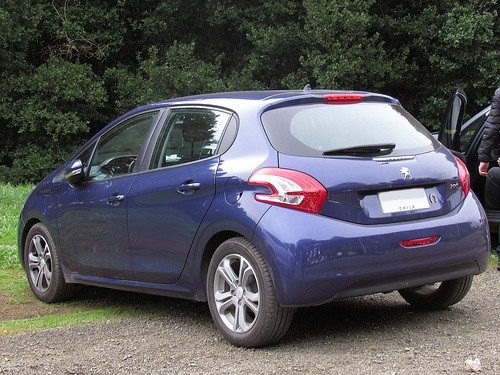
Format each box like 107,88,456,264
429,85,500,221
18,83,490,349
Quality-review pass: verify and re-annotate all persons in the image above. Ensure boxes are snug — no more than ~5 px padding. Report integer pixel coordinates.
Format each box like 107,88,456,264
478,87,500,209
